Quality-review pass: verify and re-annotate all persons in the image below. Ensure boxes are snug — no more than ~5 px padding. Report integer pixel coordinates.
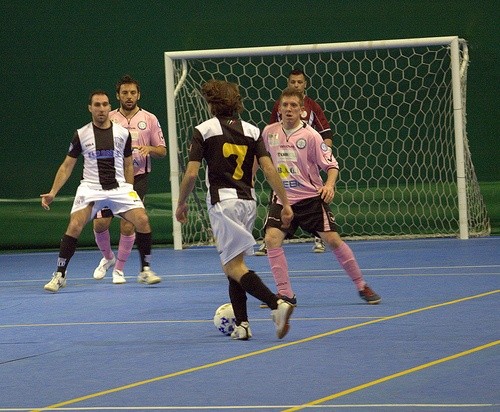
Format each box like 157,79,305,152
92,74,166,284
253,69,335,255
251,87,381,304
177,82,294,340
39,92,162,290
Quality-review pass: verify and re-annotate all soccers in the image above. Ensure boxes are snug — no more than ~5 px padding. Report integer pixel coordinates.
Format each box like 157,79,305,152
211,301,237,337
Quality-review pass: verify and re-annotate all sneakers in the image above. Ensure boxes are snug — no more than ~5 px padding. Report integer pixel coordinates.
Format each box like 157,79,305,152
231,322,253,340
137,267,161,285
44,272,66,291
271,299,294,339
255,241,268,255
312,236,326,252
93,254,116,279
259,293,297,308
112,268,126,284
358,284,382,304
